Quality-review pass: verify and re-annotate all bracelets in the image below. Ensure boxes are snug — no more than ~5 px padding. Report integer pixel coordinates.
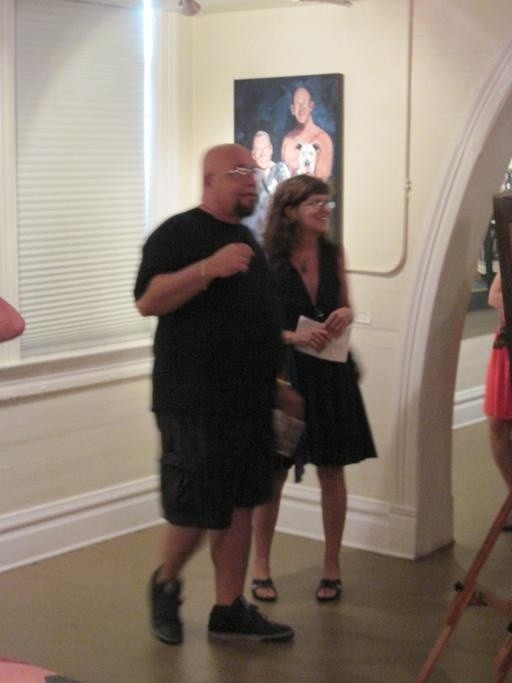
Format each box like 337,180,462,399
200,259,207,289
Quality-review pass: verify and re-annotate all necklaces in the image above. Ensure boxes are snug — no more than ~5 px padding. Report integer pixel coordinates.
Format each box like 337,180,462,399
299,257,313,272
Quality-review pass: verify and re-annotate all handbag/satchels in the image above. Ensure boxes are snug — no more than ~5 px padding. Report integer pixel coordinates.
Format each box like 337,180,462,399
272,409,305,460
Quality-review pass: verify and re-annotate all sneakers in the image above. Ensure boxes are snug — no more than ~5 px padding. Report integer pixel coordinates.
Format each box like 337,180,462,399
206,597,294,641
145,565,184,644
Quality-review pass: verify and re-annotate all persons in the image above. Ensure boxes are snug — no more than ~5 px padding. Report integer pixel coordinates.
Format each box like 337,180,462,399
240,86,334,241
251,174,378,603
134,143,298,645
483,267,512,532
0,297,25,343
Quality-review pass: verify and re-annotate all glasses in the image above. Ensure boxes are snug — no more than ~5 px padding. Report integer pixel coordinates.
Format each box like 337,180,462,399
302,199,338,210
216,166,268,177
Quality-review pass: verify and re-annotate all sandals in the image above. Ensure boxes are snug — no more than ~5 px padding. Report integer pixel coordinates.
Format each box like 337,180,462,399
251,579,277,601
316,578,341,601
